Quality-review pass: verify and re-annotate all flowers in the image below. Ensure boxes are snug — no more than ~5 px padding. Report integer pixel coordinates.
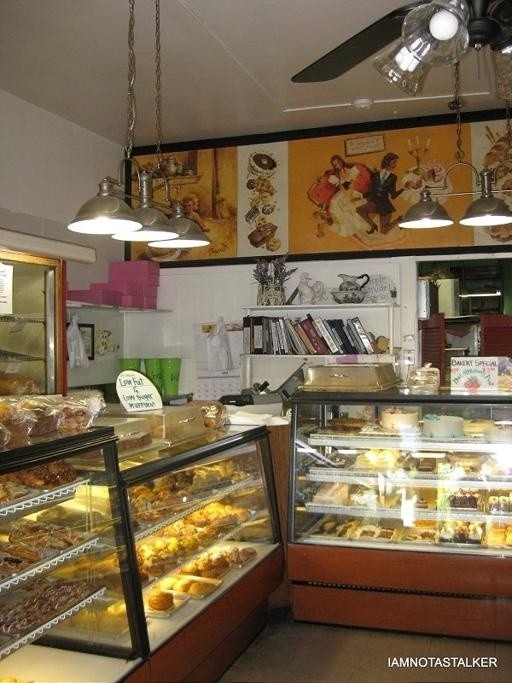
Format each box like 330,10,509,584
248,248,298,287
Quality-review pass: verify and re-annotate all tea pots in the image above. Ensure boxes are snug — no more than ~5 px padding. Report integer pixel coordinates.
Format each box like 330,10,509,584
339,273,369,290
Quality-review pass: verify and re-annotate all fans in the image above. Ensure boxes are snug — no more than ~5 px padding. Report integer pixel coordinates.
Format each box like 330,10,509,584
290,0,511,83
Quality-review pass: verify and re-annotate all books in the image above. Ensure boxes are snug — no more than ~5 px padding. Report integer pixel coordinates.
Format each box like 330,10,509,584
241,314,377,354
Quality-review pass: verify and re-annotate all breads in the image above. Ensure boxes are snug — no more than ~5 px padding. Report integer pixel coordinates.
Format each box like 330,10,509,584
1,372,88,448
1,517,86,580
1,458,78,504
155,576,217,595
72,523,216,590
180,545,258,579
182,501,253,532
129,461,253,522
149,591,173,611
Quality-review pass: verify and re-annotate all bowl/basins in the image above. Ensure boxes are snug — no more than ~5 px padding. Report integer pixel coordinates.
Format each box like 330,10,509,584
332,291,365,303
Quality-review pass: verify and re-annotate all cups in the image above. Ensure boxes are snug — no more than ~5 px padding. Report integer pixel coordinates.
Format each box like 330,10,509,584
144,359,160,392
120,358,141,373
159,358,180,396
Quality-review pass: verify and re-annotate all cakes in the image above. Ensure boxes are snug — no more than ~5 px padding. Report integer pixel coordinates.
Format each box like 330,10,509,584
422,413,465,438
378,407,420,430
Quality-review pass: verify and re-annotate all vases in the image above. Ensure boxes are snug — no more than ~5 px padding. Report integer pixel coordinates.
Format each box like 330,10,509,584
257,283,286,306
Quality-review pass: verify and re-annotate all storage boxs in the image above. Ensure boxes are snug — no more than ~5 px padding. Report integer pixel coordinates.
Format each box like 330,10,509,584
449,356,511,394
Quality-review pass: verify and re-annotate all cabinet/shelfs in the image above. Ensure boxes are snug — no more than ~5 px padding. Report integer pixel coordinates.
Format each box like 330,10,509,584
239,302,399,360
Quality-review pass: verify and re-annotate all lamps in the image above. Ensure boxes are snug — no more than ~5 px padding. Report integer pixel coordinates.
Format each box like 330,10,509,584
369,0,475,97
397,63,512,228
65,0,212,253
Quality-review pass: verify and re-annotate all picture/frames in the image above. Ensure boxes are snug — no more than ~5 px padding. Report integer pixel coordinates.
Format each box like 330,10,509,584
66,322,95,360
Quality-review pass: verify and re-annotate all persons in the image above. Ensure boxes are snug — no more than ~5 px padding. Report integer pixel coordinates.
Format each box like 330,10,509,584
181,193,210,232
210,316,235,375
356,152,409,235
316,154,368,239
